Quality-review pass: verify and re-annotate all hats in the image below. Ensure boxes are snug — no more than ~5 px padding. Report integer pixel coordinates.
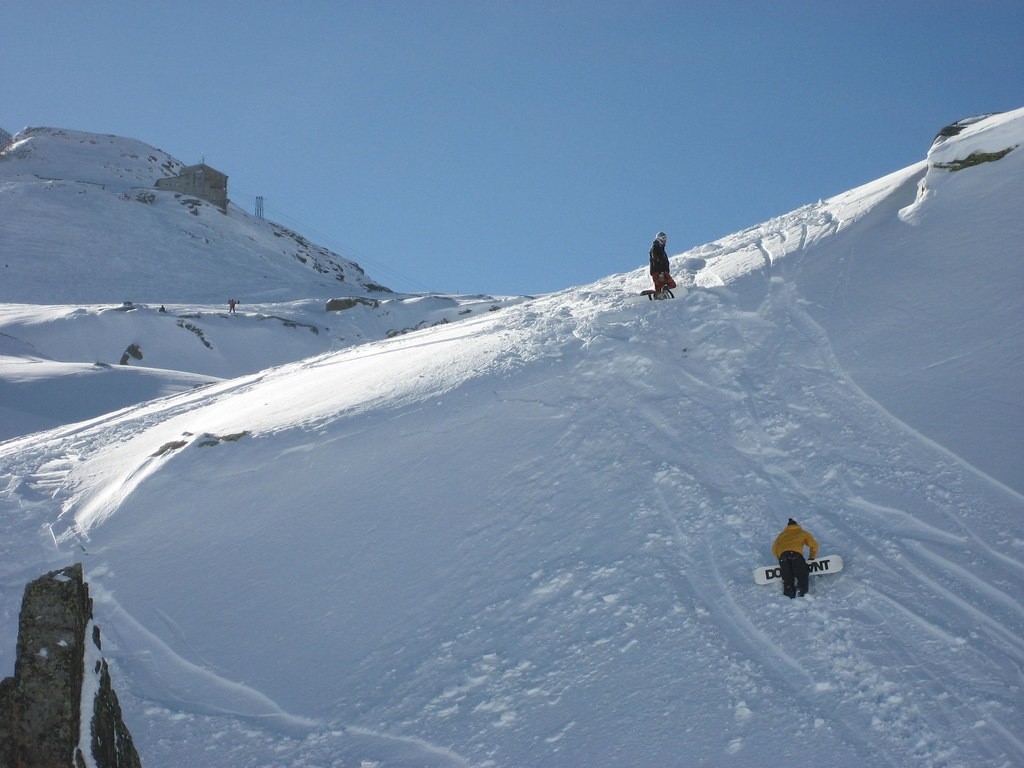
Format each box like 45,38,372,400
656,232,666,240
788,518,796,525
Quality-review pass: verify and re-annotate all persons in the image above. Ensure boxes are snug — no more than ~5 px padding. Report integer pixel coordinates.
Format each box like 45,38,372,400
649,231,676,292
773,518,818,599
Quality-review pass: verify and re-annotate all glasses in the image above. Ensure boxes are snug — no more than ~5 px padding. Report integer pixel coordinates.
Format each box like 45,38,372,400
661,236,666,241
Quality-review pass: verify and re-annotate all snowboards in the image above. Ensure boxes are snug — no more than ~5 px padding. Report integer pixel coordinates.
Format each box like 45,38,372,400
627,286,690,305
752,554,844,585
